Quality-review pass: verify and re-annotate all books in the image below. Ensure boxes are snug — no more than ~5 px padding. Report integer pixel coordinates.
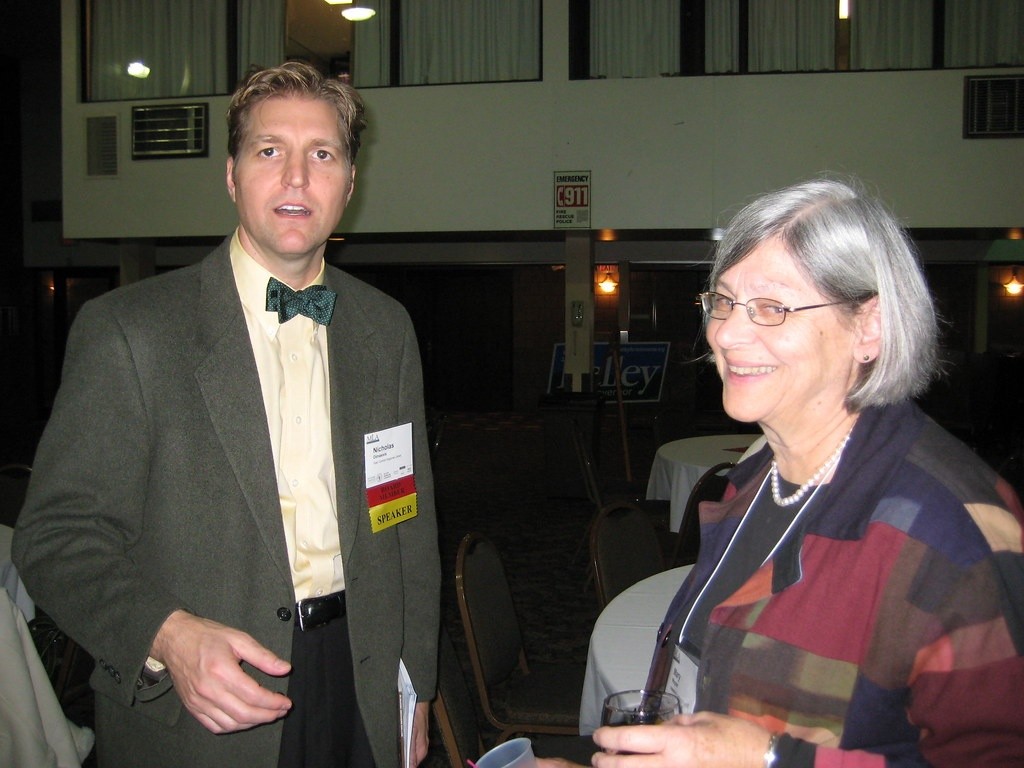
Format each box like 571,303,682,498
396,657,417,768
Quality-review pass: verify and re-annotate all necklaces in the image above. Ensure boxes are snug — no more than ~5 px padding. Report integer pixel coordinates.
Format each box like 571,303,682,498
771,419,857,506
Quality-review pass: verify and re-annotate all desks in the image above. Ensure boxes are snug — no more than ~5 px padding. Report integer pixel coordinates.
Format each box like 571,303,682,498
0,524,35,623
645,434,763,533
579,564,699,736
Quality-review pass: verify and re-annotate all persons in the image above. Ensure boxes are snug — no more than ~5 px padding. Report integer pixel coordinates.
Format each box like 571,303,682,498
536,180,1024,768
9,61,442,768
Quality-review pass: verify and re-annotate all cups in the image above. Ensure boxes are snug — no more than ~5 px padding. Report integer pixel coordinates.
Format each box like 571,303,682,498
470,737,539,768
600,688,683,755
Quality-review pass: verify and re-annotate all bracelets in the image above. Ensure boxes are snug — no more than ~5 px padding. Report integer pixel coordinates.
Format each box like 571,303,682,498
765,732,778,768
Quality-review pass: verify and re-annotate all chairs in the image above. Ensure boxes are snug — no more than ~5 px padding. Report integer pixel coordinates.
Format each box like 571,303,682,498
0,464,33,528
455,532,587,749
55,639,80,713
589,501,669,614
432,620,603,768
998,450,1024,509
670,463,735,570
570,419,646,564
652,408,696,451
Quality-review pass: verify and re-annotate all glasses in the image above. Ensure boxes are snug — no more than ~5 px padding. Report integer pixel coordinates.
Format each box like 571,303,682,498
696,292,868,326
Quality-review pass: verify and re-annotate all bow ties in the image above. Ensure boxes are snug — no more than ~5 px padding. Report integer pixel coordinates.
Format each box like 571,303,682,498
265,277,337,326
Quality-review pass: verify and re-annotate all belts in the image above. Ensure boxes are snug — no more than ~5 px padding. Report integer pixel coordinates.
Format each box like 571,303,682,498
295,590,346,632
